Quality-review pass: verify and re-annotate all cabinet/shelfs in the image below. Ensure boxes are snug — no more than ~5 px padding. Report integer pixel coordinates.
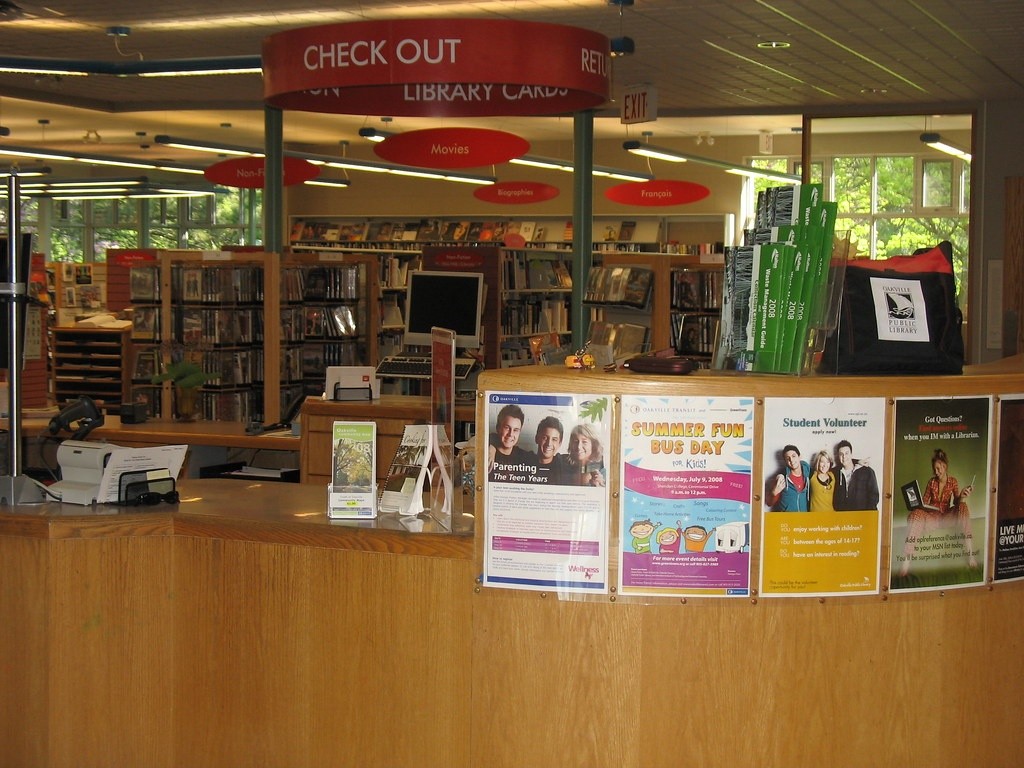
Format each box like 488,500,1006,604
500,246,697,370
132,252,379,425
603,252,725,369
47,318,133,425
293,245,423,394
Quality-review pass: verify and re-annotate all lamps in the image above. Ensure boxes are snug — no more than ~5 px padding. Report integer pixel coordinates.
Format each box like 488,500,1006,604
919,114,971,161
622,127,803,186
360,117,656,183
607,0,637,61
0,52,353,201
152,132,498,185
758,128,774,155
694,131,716,147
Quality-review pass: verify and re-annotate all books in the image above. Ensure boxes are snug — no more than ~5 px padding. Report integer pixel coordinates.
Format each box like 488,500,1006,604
129,218,725,425
73,315,132,329
724,183,837,373
379,425,434,515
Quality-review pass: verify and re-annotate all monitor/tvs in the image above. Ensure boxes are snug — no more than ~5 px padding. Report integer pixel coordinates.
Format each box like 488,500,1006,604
404,270,484,349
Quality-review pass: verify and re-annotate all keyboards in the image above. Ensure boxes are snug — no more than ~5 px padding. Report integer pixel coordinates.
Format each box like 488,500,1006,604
374,355,476,380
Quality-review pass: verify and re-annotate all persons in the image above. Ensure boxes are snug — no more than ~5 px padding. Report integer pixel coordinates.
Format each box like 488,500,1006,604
443,220,469,241
766,440,880,512
493,222,504,241
900,449,978,576
488,404,607,487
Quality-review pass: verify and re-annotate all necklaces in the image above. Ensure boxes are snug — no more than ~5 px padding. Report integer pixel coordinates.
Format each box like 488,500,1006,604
821,476,830,487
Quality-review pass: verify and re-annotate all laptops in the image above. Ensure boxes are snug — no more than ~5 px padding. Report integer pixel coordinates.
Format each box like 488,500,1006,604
902,480,940,512
